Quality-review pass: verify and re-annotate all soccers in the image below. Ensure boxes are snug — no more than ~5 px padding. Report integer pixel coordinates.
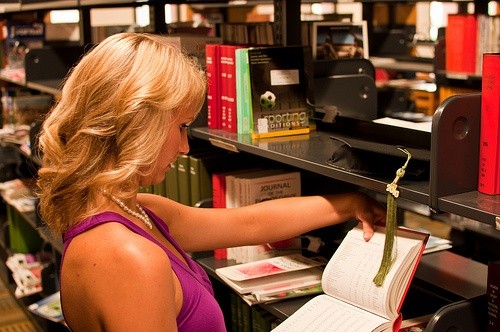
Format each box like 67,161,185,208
259,90,277,109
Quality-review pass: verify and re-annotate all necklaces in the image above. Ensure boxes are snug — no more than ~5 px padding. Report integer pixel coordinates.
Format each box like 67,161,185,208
93,184,153,231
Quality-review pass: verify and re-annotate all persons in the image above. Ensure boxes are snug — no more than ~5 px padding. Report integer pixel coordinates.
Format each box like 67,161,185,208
37,31,386,332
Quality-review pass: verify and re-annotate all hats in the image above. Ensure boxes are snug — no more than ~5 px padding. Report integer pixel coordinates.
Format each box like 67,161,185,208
325,122,431,286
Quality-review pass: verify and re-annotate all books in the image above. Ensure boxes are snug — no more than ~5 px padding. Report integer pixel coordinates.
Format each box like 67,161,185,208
0,0,500,332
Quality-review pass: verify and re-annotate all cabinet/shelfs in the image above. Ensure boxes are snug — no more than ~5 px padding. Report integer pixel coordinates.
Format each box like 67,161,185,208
1,0,500,332
